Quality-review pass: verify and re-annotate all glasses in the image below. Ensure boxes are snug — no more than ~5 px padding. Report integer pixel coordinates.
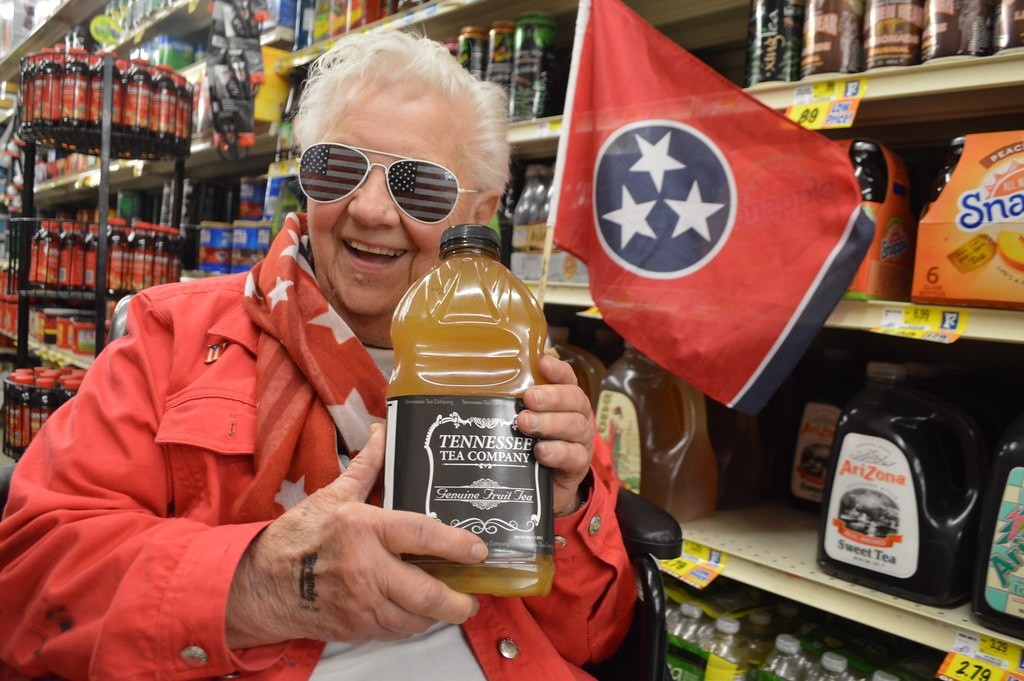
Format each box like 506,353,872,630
294,141,485,226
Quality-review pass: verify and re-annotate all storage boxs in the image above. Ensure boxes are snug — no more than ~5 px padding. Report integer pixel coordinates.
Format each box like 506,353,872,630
836,138,915,301
909,129,1024,311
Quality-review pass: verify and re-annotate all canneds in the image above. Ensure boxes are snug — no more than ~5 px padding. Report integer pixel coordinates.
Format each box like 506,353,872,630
485,20,516,102
509,11,561,123
444,40,458,60
456,26,489,81
748,0,1024,91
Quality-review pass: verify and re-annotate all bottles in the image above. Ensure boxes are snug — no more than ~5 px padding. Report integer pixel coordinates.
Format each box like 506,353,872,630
513,168,553,223
6,367,85,455
383,224,553,596
927,137,966,210
852,142,885,202
555,326,1024,681
30,217,180,296
23,43,192,157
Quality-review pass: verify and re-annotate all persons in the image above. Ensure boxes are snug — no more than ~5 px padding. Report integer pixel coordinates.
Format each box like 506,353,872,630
0,32,638,680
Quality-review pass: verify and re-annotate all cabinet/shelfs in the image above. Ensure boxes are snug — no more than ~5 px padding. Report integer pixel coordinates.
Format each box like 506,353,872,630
2,50,195,462
1,1,287,375
268,0,1024,681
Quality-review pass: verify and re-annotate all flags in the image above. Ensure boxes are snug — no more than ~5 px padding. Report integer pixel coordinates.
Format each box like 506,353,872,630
545,1,875,413
